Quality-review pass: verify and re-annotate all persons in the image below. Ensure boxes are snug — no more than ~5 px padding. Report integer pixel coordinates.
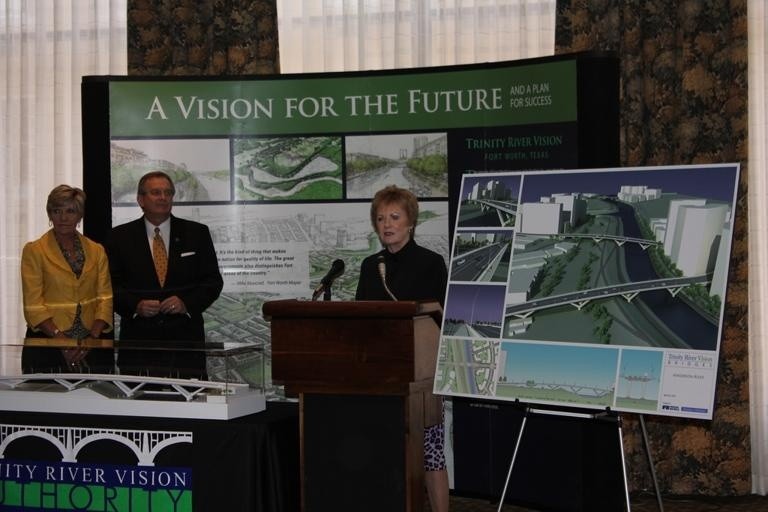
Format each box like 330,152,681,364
102,172,223,389
21,185,115,387
355,186,452,511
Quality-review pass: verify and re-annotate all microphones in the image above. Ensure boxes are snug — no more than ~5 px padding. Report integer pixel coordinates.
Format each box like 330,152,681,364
377,255,398,301
315,259,345,295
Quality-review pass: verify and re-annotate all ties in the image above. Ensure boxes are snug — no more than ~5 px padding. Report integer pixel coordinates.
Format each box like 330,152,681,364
153,225,171,290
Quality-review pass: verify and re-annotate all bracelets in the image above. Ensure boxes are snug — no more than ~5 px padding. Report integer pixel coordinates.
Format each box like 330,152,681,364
52,328,65,338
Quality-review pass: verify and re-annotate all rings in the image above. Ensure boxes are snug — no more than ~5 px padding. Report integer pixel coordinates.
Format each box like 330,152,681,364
81,350,84,354
172,305,175,310
168,305,172,312
149,311,153,315
64,349,68,352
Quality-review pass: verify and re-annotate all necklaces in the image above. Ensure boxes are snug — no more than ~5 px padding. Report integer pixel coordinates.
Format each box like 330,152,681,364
61,248,76,257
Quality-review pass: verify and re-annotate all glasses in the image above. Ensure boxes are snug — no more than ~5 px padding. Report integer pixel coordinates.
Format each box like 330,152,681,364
138,189,174,197
